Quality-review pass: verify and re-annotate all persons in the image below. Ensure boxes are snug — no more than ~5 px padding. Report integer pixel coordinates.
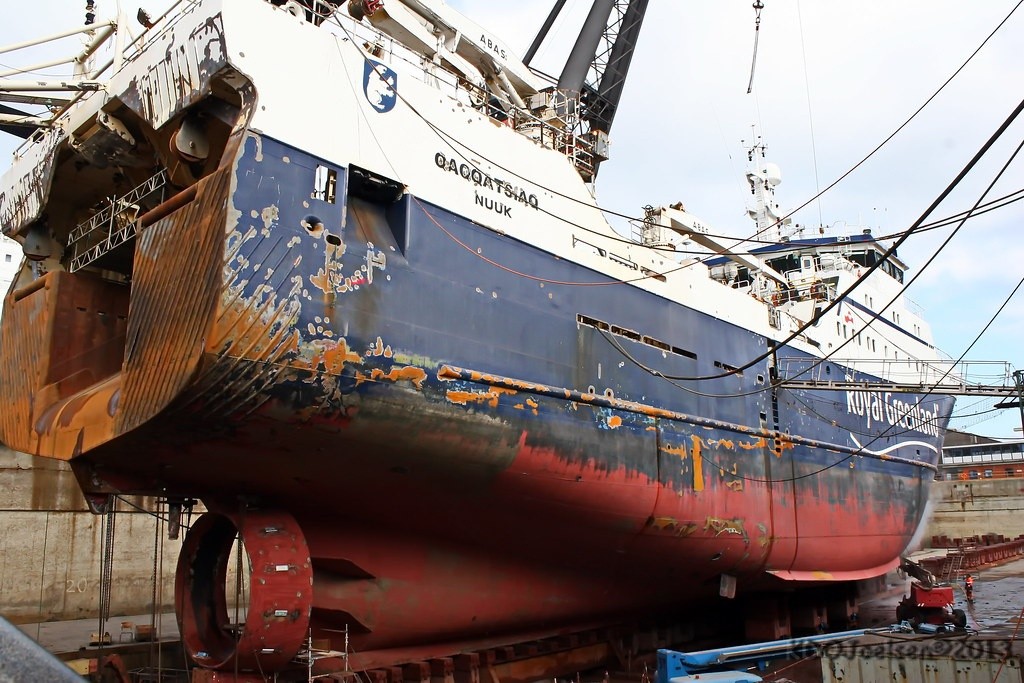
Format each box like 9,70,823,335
965,574,974,603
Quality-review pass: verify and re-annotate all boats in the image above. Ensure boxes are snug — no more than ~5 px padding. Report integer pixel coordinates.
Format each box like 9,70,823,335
0,1,965,683
